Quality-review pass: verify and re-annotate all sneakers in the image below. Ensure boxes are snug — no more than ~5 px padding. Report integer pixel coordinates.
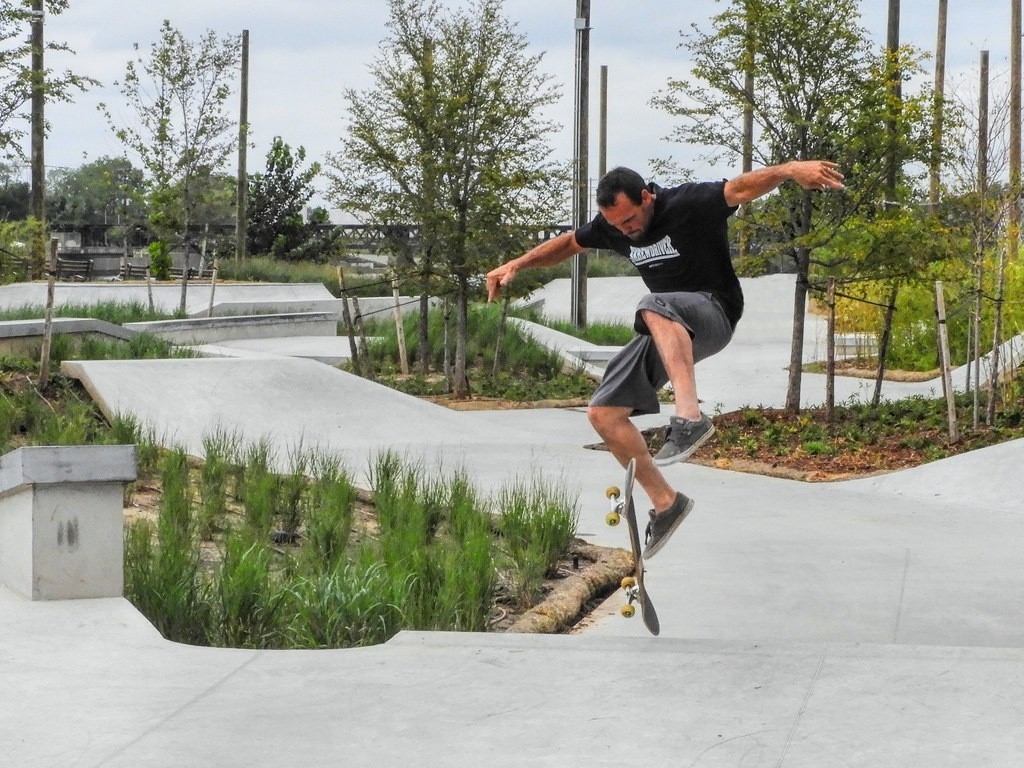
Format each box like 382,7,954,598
642,492,695,560
652,411,715,466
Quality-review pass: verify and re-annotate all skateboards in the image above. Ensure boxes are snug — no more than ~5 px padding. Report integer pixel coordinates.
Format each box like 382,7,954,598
602,456,664,637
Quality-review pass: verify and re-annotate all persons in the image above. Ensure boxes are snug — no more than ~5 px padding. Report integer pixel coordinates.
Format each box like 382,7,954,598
483,159,846,562
